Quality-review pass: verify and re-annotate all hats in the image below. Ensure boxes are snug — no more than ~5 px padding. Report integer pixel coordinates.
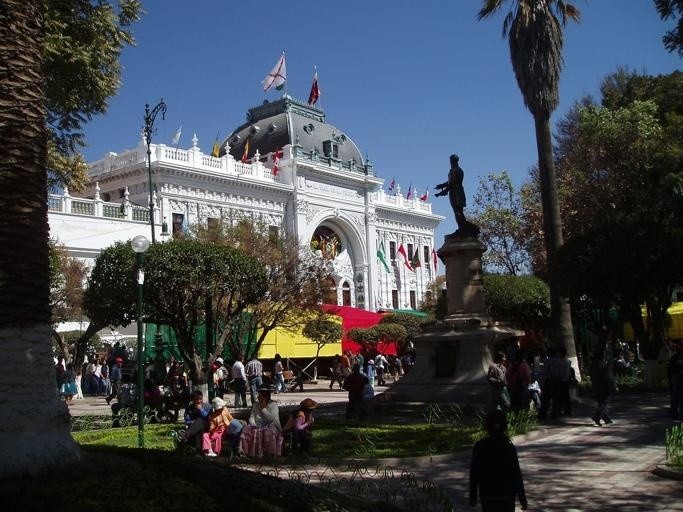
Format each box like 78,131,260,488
227,418,244,434
216,357,224,365
115,357,124,365
212,397,229,413
300,398,320,411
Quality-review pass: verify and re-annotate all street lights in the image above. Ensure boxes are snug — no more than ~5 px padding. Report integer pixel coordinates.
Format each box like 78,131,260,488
130,234,149,449
159,214,172,242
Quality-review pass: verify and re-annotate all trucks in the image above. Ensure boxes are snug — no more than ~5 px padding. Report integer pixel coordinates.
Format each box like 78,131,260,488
610,297,682,350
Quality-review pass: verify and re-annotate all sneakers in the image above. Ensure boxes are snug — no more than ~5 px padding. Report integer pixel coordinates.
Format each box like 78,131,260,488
593,415,612,427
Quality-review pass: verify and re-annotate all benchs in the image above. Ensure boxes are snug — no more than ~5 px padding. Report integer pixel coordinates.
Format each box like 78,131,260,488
262,368,297,385
178,423,315,460
120,394,181,426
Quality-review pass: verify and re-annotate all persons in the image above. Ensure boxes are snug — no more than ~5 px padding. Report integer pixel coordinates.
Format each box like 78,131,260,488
469,409,527,512
434,154,466,228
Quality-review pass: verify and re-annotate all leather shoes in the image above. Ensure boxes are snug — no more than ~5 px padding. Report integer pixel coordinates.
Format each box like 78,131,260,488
105,398,110,405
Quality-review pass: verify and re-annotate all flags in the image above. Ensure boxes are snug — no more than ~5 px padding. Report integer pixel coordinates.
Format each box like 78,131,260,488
421,188,429,201
262,53,287,93
407,186,412,200
412,251,421,273
390,177,395,190
377,243,392,274
431,249,437,272
308,67,318,105
399,244,413,272
170,123,280,178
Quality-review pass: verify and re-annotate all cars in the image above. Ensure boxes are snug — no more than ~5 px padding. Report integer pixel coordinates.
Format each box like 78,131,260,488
117,359,136,382
